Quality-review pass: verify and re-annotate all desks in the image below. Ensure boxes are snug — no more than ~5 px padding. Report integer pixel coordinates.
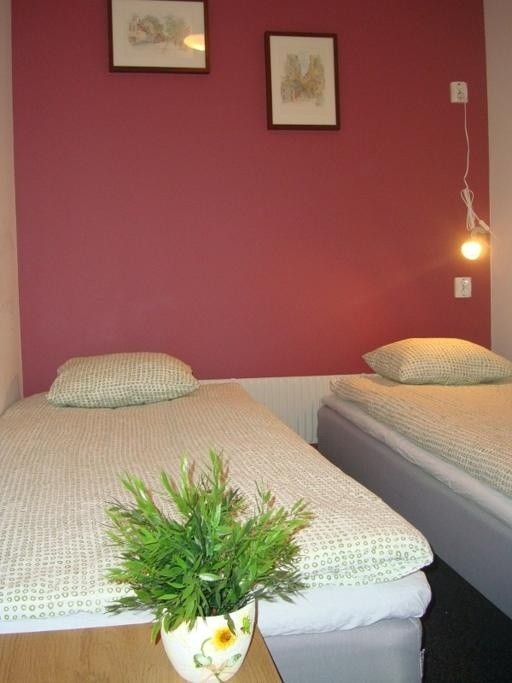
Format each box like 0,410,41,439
0,615,283,682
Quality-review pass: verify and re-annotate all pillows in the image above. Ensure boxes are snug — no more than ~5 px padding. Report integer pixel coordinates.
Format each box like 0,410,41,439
46,350,200,411
362,338,510,386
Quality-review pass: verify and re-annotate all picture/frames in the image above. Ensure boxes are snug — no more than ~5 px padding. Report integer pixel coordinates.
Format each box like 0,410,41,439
108,1,211,75
264,29,342,133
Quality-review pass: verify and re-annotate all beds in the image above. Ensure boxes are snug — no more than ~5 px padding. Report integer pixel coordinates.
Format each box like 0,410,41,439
0,379,436,683
319,373,511,682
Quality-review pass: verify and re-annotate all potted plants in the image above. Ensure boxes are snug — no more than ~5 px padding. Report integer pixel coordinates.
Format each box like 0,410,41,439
101,443,320,682
